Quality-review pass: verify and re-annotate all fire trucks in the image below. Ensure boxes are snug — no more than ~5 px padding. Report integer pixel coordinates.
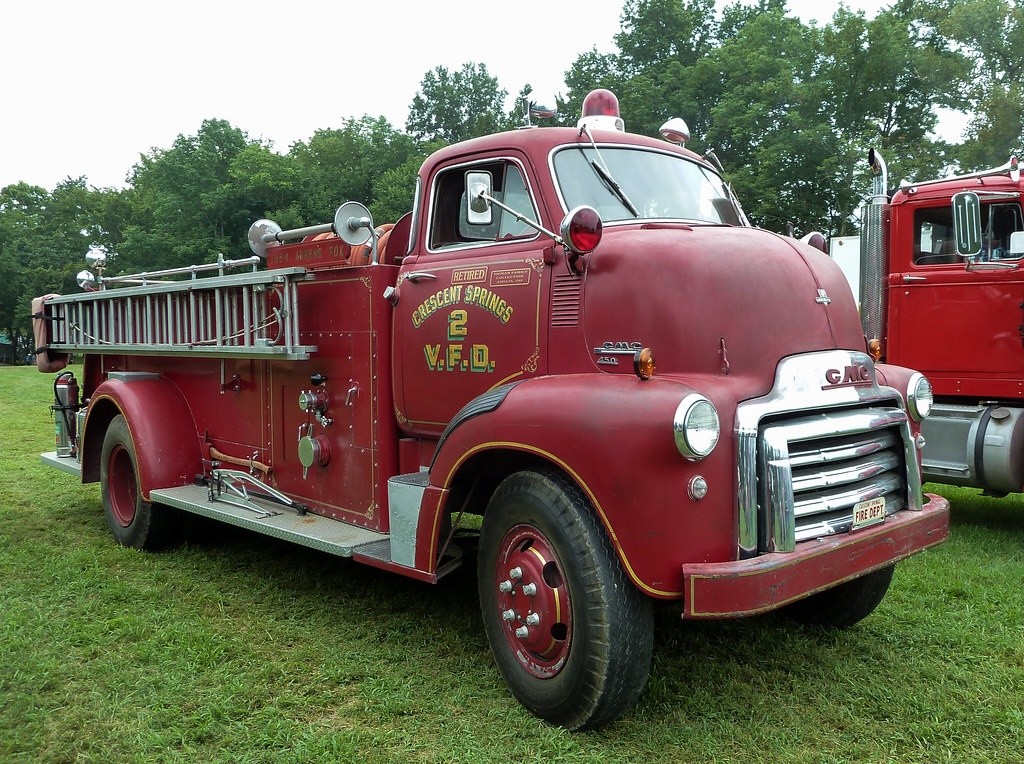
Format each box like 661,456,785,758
797,145,1024,499
31,88,950,732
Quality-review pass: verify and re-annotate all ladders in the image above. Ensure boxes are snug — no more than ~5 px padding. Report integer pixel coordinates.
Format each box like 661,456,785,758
49,266,319,360
